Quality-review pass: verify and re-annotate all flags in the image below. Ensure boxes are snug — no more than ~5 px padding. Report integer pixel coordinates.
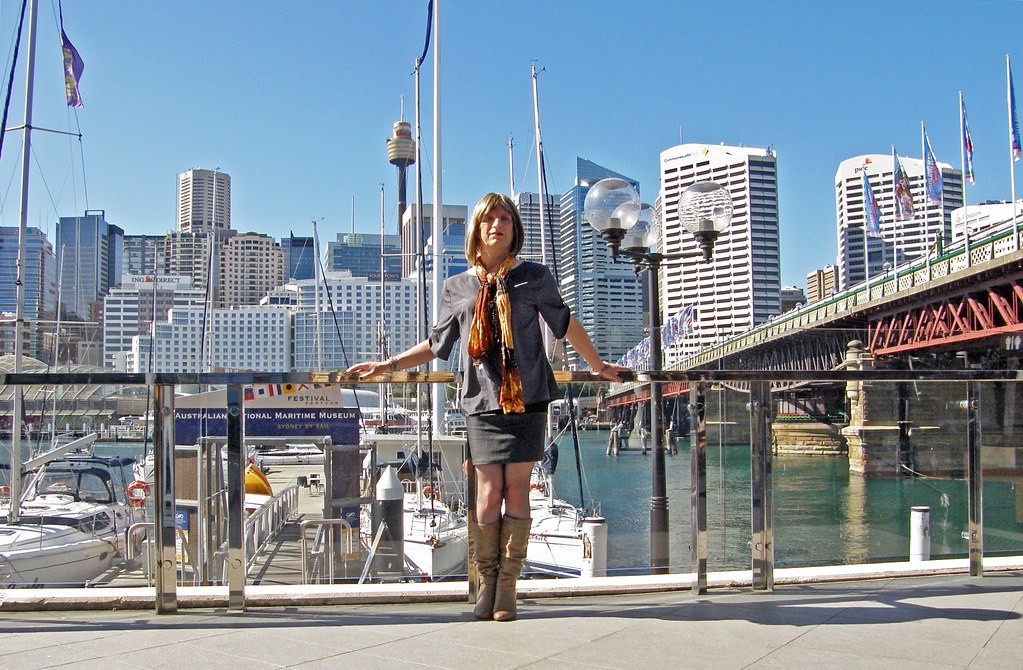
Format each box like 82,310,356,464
864,63,1022,235
61,31,84,107
619,308,692,367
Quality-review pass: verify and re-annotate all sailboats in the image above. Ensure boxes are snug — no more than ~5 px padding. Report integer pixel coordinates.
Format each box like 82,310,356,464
0,0,609,590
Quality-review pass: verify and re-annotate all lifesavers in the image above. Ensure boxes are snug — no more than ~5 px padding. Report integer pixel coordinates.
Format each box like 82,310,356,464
530,484,545,494
128,479,151,506
0,485,11,498
423,486,440,499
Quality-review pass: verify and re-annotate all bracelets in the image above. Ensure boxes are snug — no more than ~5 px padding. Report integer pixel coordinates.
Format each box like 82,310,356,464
387,357,400,371
591,361,609,375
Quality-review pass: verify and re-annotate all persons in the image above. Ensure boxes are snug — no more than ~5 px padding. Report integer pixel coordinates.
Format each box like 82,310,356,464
346,193,636,623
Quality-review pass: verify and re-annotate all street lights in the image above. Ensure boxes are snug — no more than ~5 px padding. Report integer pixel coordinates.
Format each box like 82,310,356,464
582,177,734,575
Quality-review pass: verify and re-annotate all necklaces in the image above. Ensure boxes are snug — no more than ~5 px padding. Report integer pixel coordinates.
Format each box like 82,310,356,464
475,267,510,343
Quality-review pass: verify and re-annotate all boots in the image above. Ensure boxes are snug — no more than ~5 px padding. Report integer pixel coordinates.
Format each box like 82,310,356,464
470,515,502,620
491,514,534,621
